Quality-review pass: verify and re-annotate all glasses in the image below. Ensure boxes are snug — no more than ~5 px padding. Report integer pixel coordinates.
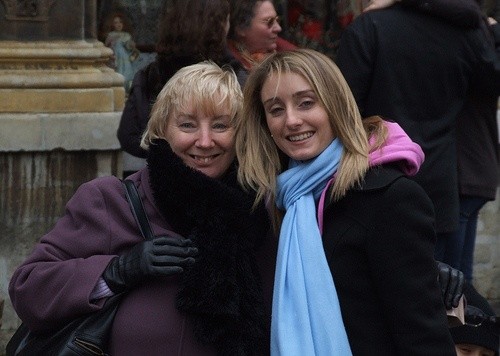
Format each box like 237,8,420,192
251,16,280,28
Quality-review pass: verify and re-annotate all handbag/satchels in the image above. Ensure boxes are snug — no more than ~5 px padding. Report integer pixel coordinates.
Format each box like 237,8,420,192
5,179,155,356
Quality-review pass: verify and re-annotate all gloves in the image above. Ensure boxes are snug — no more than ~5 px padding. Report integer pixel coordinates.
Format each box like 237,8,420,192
434,258,465,307
103,236,197,295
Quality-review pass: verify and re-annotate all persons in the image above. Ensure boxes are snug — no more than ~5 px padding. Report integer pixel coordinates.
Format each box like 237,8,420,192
435,261,500,356
235,51,457,356
328,0,500,282
118,0,323,158
104,11,141,96
9,59,464,356
